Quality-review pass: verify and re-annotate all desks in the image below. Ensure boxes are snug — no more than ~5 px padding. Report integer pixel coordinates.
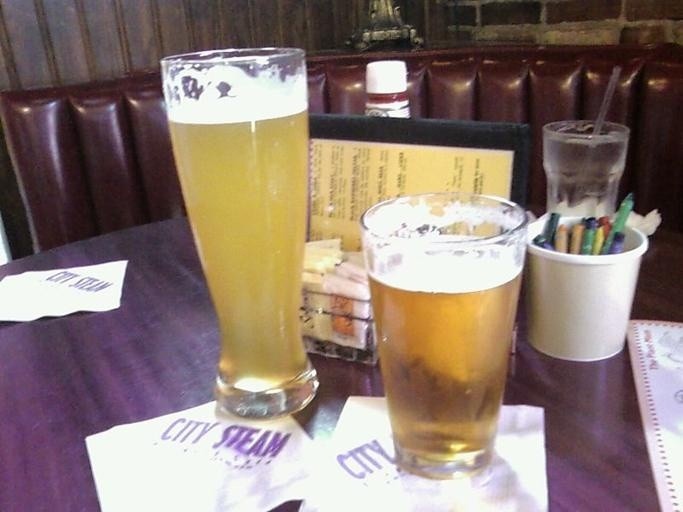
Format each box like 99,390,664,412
2,218,683,511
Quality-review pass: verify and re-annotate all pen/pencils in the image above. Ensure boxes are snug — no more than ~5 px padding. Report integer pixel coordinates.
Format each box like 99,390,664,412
534,191,637,255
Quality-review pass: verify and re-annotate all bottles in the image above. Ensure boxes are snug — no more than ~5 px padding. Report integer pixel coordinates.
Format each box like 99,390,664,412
362,61,412,121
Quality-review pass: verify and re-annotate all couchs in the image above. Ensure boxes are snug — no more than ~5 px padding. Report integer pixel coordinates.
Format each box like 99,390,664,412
2,46,681,310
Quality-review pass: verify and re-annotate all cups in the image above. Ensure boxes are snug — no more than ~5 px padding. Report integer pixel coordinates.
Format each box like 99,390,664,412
158,45,321,420
360,191,537,490
525,216,650,363
542,120,631,219
